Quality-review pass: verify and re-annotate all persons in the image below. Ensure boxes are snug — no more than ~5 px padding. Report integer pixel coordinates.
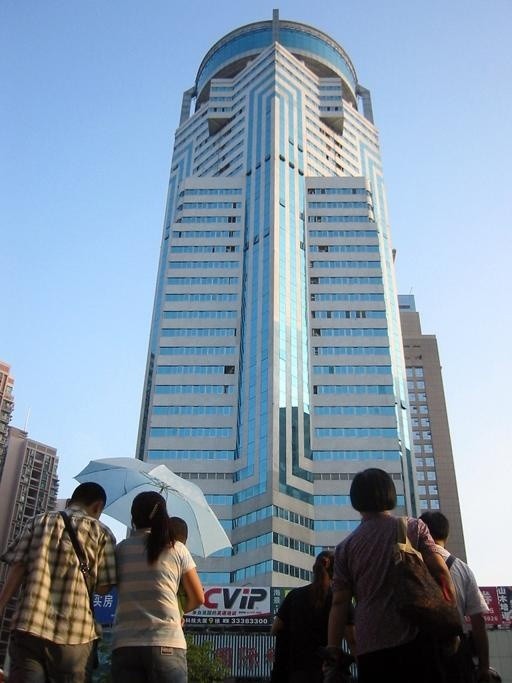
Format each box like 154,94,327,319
266,548,358,682
417,510,492,683
320,466,462,682
1,481,118,682
167,516,189,625
109,490,206,682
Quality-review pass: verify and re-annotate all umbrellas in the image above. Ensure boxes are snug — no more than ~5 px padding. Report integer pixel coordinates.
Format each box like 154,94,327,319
72,455,235,560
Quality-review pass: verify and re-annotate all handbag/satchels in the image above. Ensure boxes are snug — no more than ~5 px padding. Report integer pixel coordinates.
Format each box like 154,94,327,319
392,544,465,638
92,619,103,669
